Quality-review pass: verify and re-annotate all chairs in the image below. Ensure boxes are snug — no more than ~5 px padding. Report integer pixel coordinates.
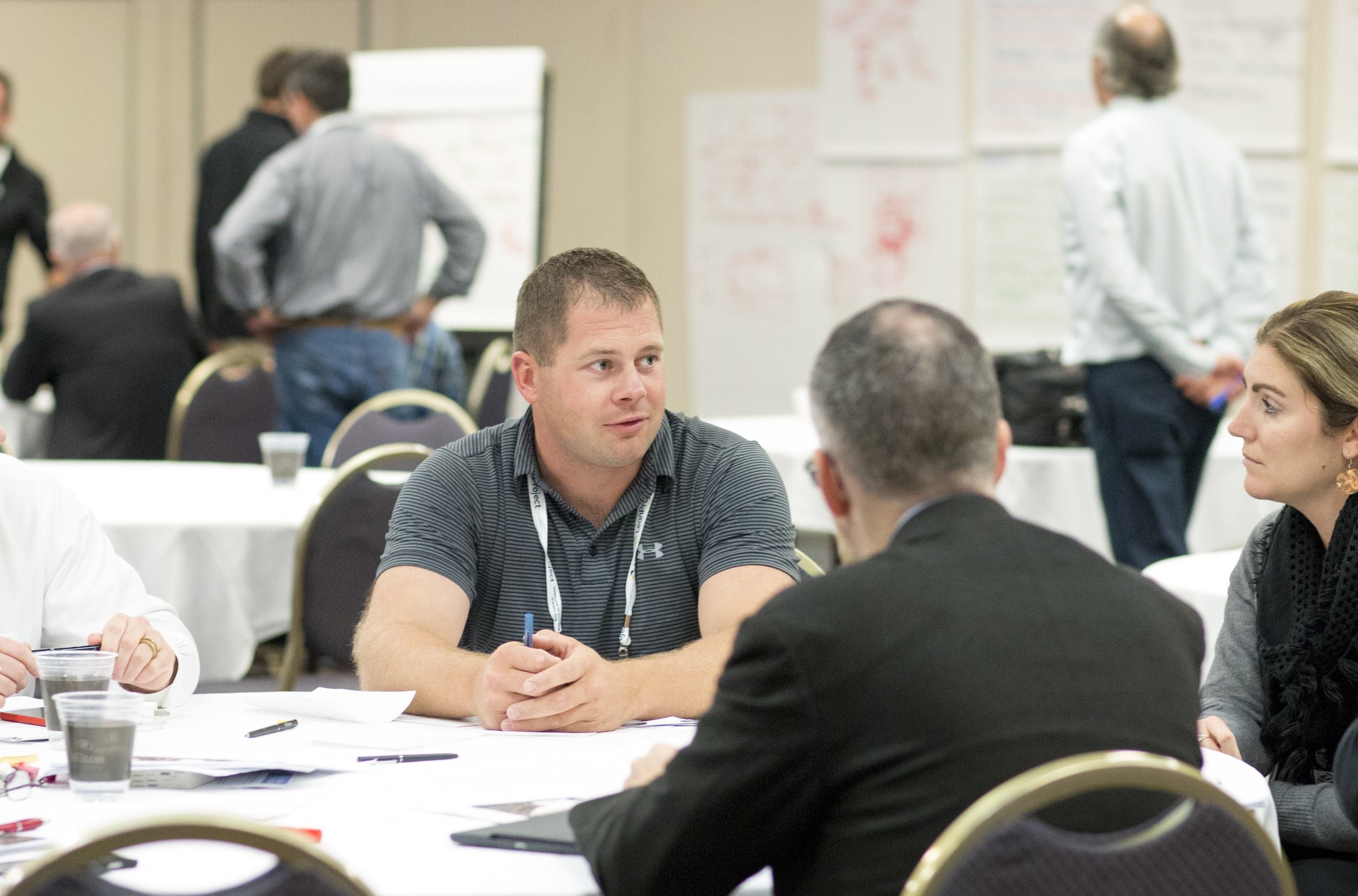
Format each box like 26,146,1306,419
466,336,514,428
907,747,1297,895
256,443,433,686
321,388,479,470
164,346,276,463
0,814,370,895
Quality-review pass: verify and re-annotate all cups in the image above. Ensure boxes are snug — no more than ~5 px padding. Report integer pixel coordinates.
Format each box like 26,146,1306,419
255,429,312,490
37,650,119,742
51,687,146,794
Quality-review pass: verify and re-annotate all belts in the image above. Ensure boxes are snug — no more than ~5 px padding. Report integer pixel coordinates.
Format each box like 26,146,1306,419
289,313,407,335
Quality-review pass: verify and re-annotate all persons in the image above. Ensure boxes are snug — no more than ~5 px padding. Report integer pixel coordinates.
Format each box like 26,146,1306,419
567,297,1209,896
1178,290,1357,896
341,243,800,742
0,417,202,709
1045,4,1272,577
0,42,491,473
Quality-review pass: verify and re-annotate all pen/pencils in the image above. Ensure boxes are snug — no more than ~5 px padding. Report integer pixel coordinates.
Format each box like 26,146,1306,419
522,611,536,649
359,750,460,761
27,643,103,655
1206,372,1244,412
245,718,299,739
0,817,44,834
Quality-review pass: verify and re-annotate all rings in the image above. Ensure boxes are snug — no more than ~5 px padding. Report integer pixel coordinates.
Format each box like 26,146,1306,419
140,638,157,659
1196,734,1212,742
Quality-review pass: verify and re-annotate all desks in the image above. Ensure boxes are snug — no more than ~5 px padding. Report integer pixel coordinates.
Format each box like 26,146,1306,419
0,688,1281,896
22,457,413,682
